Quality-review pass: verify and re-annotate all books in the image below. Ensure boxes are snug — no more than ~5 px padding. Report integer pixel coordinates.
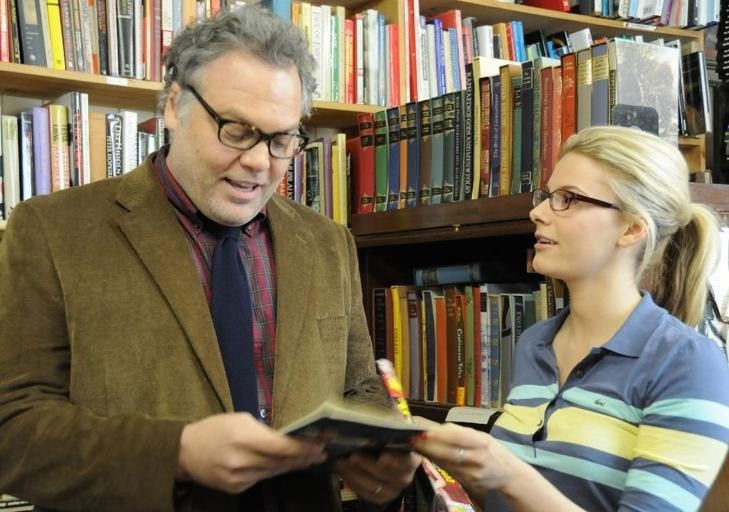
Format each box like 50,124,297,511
358,37,680,213
1,92,171,220
260,1,723,138
276,134,348,231
277,401,426,467
370,263,570,412
1,1,249,84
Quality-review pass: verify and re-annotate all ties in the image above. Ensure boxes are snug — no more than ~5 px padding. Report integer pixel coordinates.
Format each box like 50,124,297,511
209,226,260,422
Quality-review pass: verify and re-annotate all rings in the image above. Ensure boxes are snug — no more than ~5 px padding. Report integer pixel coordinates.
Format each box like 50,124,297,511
458,447,465,466
373,485,386,495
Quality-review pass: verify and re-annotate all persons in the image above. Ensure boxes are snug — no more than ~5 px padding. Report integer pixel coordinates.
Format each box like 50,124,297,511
409,126,728,512
1,0,422,512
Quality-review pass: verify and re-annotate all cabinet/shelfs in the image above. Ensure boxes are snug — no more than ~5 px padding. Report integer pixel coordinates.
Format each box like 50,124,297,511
0,1,728,226
342,181,728,435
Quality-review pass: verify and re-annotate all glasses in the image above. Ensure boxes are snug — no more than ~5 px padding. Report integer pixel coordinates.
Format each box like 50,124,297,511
532,184,620,212
187,85,310,160
